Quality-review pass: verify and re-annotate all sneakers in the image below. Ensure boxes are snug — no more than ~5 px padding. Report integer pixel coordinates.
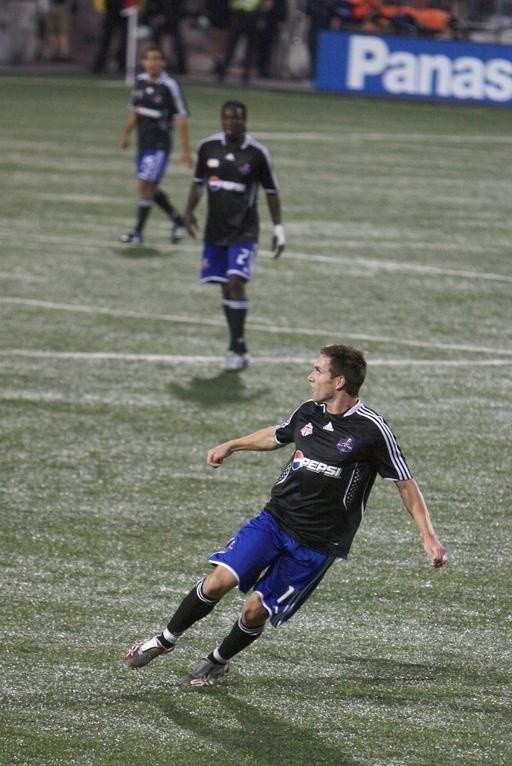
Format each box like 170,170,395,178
182,654,229,688
119,232,146,244
171,218,187,245
124,634,176,669
221,349,251,370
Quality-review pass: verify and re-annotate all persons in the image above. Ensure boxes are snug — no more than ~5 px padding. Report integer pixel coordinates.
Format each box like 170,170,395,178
182,99,286,369
116,43,195,245
29,1,470,90
120,342,451,689
